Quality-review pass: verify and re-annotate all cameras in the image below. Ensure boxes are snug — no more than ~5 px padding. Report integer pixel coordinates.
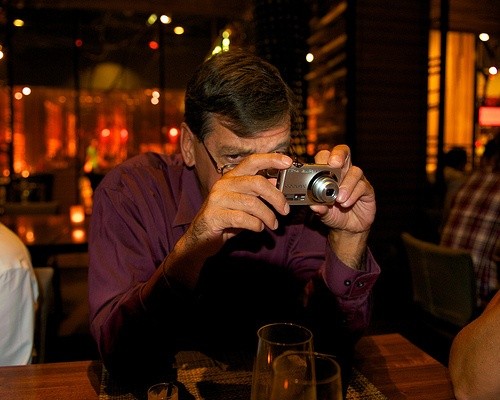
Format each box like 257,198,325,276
277,163,341,205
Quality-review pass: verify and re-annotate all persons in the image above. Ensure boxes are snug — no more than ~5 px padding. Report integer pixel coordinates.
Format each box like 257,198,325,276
331,125,500,400
87,50,381,383
0,222,39,367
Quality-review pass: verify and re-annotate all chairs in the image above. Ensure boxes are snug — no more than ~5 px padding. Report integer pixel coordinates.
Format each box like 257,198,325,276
400,233,476,325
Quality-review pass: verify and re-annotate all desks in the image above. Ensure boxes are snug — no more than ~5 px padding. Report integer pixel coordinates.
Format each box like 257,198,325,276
0,334,454,400
0,212,88,362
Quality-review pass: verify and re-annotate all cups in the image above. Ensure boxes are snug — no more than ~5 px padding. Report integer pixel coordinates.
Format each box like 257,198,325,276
272,351,343,400
147,383,178,400
251,323,317,400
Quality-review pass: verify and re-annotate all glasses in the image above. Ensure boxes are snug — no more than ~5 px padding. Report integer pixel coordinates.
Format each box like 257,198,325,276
193,128,298,178
